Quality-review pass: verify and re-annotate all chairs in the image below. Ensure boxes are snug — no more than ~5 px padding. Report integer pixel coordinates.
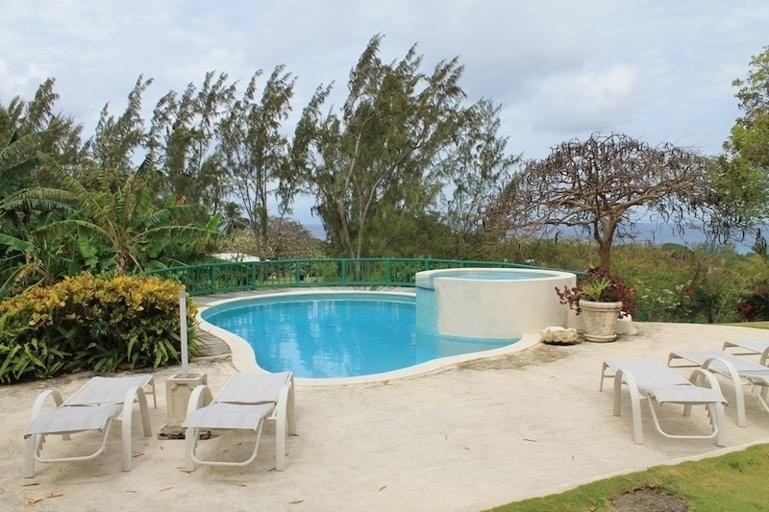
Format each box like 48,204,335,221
22,373,157,479
600,336,769,447
182,369,297,472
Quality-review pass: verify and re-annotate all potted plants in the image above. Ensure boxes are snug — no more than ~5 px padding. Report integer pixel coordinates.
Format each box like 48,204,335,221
554,271,636,342
